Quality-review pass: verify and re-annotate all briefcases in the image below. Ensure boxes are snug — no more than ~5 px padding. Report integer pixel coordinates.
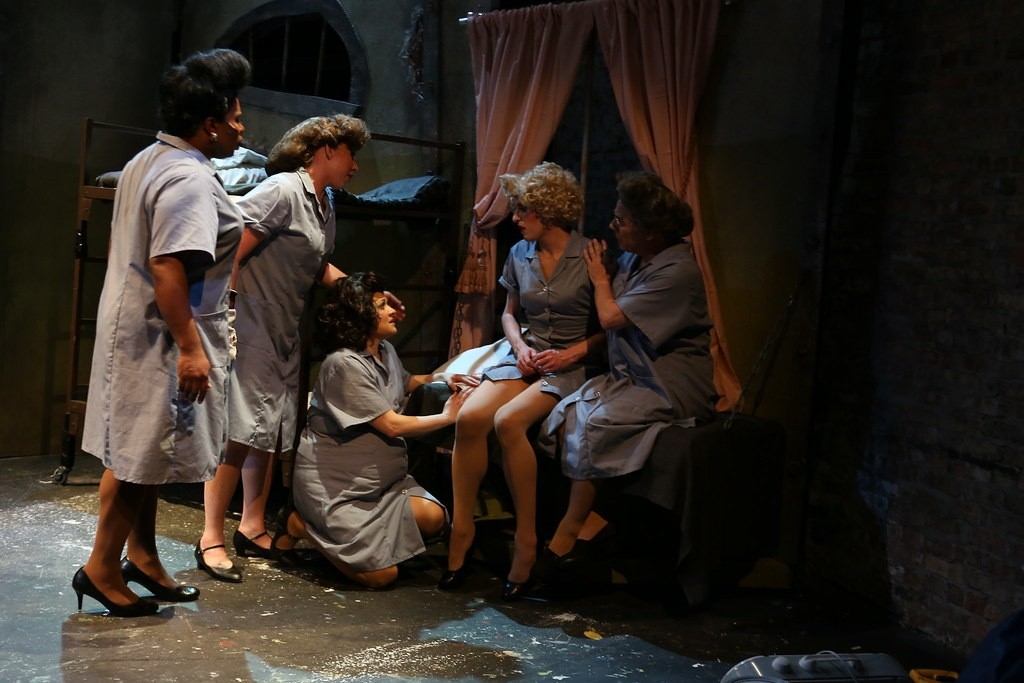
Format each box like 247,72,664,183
720,648,911,683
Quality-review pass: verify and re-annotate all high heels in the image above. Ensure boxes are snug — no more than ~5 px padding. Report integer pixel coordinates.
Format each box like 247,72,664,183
271,508,302,567
233,529,276,558
195,538,243,582
120,555,201,601
440,541,471,589
501,563,535,601
72,566,158,617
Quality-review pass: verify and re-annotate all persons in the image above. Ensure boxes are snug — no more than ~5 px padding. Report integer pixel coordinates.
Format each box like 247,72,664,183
436,160,608,607
270,271,482,589
541,168,720,578
194,111,406,582
72,48,253,619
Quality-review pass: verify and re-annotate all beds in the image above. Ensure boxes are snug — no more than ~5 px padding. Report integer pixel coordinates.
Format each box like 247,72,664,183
408,392,785,618
52,118,464,484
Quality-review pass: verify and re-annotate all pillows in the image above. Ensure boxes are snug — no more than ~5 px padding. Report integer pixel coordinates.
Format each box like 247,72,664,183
368,175,455,201
220,166,269,185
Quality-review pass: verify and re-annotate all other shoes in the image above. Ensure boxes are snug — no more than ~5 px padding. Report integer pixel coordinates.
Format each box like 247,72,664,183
538,545,573,573
